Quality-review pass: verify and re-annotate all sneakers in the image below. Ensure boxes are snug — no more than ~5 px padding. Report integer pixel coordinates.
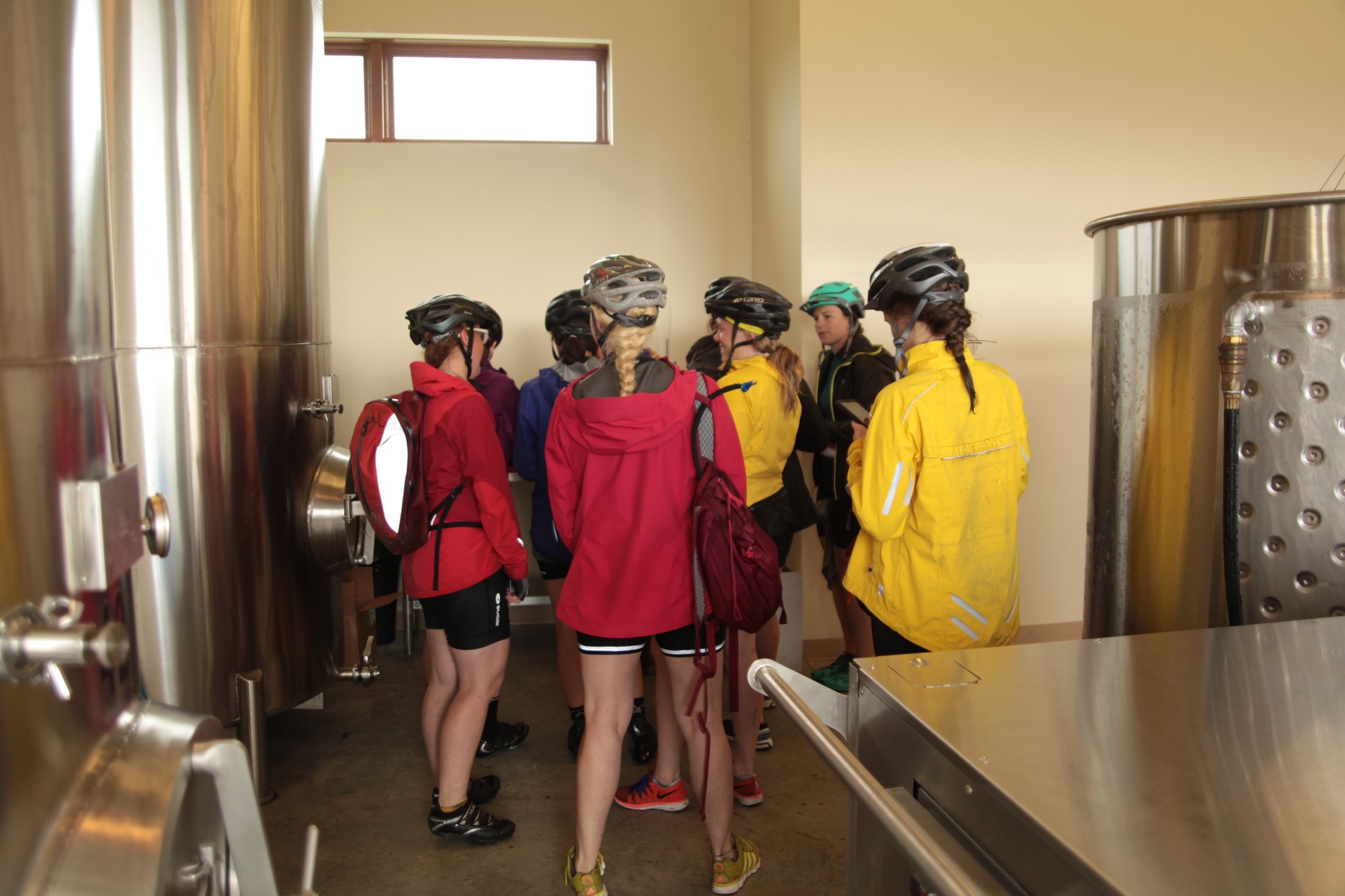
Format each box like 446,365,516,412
712,833,761,894
811,651,855,682
567,719,585,758
723,719,773,750
628,711,657,764
565,845,608,896
614,768,689,812
732,759,763,806
763,696,776,709
428,800,516,845
476,722,529,758
821,670,850,691
432,775,501,807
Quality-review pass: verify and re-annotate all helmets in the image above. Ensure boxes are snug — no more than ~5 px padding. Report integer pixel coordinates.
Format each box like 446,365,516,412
798,281,865,318
545,289,593,336
475,300,503,349
405,294,485,345
704,276,793,340
862,243,969,311
581,254,667,311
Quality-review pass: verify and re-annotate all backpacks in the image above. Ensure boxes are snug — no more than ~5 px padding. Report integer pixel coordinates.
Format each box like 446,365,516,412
350,389,482,556
691,371,787,678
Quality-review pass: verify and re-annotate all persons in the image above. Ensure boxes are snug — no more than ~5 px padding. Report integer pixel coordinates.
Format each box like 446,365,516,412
612,276,856,812
811,280,895,692
395,296,520,845
847,243,1031,655
469,299,527,758
513,288,657,765
543,254,762,896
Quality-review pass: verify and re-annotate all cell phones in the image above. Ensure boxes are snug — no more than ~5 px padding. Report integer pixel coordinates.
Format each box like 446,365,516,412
836,398,872,428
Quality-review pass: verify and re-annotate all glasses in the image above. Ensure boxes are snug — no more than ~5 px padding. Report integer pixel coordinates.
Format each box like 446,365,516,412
457,327,489,344
